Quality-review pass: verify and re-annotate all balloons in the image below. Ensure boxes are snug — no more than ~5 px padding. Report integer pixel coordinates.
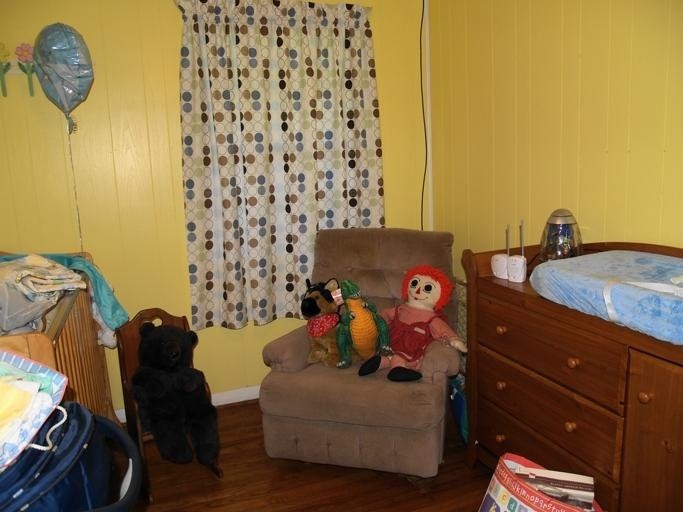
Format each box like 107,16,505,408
31,23,94,120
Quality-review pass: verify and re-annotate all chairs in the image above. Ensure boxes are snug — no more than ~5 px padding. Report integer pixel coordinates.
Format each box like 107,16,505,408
259,228,456,483
116,308,224,503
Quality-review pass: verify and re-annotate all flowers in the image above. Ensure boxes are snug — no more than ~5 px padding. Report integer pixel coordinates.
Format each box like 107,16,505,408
16,43,37,97
0,43,11,98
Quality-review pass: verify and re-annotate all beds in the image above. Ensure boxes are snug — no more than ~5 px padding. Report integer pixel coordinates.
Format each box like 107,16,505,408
0,253,122,425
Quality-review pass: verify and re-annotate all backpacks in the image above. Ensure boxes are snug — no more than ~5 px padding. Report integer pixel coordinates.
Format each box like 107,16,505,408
0,400,149,512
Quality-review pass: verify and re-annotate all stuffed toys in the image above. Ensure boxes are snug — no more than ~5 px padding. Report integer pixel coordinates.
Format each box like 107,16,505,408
301,277,363,368
334,280,390,371
126,320,220,468
354,266,470,383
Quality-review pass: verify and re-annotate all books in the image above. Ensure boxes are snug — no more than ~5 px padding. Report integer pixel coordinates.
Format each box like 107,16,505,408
513,465,595,511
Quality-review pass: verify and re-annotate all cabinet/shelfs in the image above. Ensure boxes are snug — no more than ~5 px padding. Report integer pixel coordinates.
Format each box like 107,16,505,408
460,241,683,512
